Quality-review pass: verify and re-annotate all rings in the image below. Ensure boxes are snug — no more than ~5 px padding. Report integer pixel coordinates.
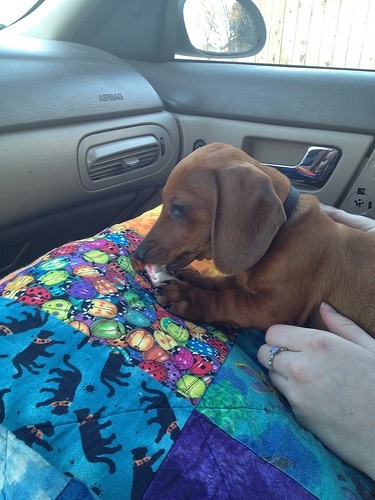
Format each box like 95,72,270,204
266,346,289,372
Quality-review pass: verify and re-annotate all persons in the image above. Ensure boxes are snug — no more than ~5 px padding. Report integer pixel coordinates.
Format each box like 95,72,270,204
256,204,374,480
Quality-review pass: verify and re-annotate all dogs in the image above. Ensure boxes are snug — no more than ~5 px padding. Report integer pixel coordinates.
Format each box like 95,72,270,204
135,142,374,339
